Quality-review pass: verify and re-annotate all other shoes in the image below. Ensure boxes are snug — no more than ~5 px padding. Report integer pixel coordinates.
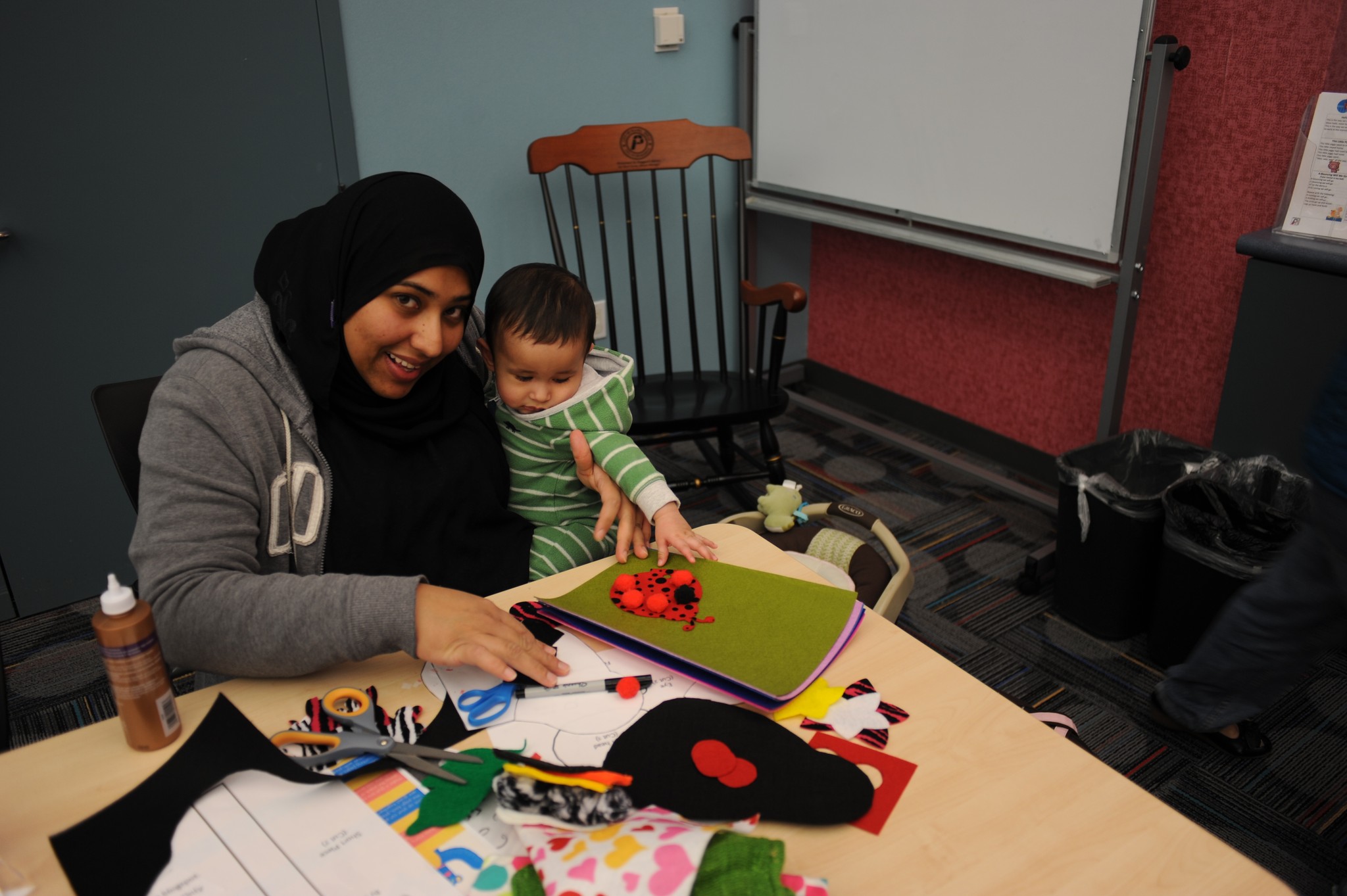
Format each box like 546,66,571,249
1140,691,1273,756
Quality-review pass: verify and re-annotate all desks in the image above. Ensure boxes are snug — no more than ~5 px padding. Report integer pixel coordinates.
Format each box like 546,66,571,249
1212,225,1347,495
0,524,1303,895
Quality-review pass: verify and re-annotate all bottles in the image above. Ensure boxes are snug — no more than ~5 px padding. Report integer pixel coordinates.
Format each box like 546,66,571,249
89,572,183,754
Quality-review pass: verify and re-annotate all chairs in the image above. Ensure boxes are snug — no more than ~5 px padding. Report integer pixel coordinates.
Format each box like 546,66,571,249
525,118,808,523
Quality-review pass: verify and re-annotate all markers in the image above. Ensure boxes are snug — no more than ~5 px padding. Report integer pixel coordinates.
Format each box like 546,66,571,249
515,673,653,699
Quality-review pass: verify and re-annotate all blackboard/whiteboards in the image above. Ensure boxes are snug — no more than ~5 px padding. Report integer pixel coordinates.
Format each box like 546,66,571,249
751,0,1157,265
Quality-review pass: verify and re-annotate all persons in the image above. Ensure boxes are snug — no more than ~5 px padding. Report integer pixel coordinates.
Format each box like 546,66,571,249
125,169,654,687
485,260,721,586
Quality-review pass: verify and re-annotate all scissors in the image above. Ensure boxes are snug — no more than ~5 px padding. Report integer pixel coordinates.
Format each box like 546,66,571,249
457,674,526,726
267,687,484,785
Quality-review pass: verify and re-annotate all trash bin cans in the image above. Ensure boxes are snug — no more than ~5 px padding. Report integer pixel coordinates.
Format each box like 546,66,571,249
1055,426,1229,641
1148,457,1316,670
